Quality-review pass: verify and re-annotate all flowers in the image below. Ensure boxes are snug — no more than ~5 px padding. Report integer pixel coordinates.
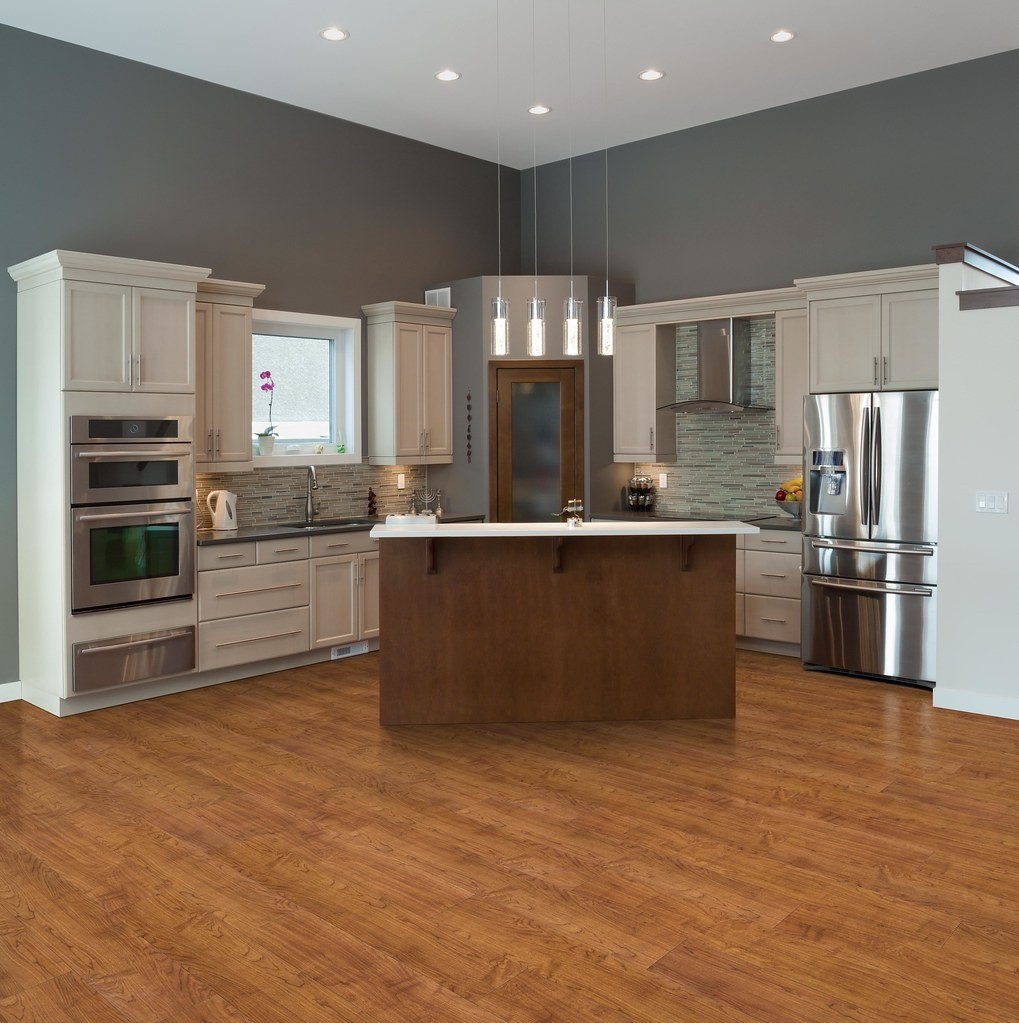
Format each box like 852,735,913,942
254,370,279,437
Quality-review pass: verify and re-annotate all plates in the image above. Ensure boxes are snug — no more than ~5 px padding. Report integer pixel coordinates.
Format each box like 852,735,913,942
775,499,802,522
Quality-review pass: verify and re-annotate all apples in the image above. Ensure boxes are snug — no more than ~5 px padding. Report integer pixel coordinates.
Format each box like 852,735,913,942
775,490,797,501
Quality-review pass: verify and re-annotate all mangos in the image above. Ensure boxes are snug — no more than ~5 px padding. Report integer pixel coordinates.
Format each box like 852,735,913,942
796,491,802,501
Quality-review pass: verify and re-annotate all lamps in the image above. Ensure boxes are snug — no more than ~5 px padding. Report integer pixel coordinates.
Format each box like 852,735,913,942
596,0,617,356
564,0,584,356
491,0,512,356
524,0,545,357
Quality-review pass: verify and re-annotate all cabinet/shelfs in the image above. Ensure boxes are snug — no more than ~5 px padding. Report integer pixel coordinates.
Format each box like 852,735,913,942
610,322,678,462
9,247,459,474
735,528,804,659
805,276,942,394
196,528,382,674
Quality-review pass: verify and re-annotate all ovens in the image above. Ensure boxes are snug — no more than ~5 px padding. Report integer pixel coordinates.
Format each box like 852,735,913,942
70,415,195,694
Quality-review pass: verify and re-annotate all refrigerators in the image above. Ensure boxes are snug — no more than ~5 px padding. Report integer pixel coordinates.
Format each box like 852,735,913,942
800,390,939,691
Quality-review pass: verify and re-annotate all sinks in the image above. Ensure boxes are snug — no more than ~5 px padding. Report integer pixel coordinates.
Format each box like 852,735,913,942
278,519,367,529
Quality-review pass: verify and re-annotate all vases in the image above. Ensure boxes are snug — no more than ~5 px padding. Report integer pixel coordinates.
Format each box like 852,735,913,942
258,437,275,454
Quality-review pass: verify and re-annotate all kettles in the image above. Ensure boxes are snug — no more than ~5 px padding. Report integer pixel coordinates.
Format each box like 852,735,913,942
206,490,239,530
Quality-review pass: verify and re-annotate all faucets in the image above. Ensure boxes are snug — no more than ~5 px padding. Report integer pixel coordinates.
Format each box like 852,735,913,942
305,465,321,522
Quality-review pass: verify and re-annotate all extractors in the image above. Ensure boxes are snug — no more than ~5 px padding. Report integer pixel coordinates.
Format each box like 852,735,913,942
656,317,775,414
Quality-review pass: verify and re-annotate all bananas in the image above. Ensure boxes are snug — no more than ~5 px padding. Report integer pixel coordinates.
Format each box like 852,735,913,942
781,478,802,492
